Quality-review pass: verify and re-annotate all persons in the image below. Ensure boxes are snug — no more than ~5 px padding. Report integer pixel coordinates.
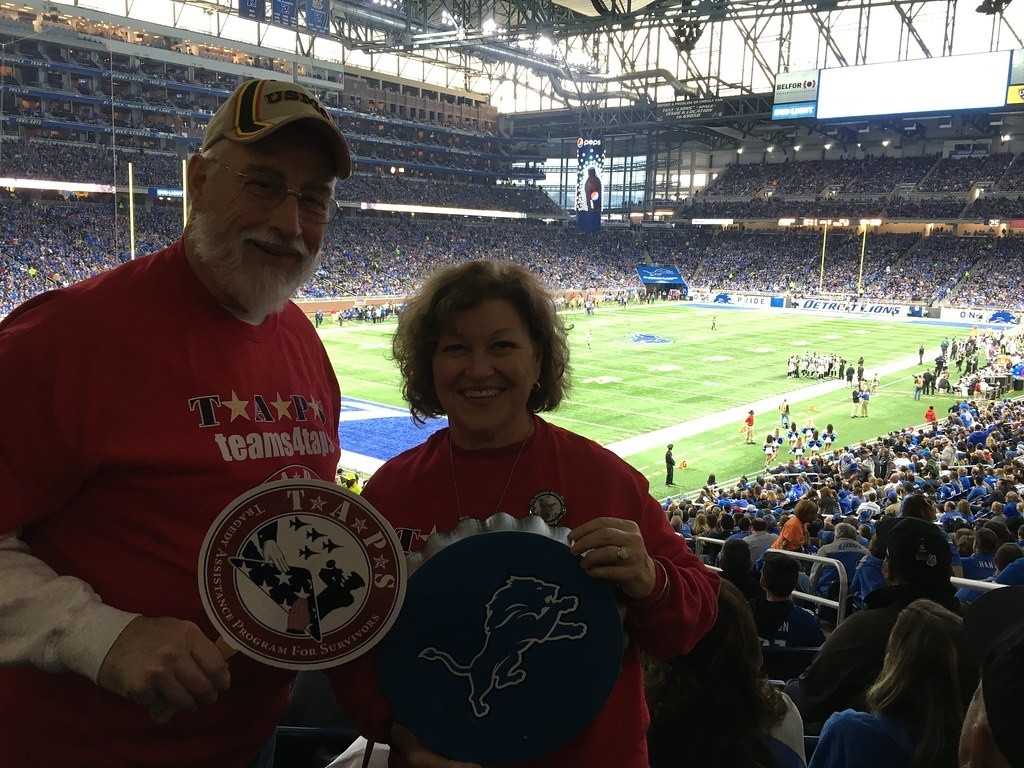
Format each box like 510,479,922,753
846,356,880,419
640,497,1024,768
0,80,351,768
404,86,670,315
711,317,716,331
914,327,1024,401
695,397,1024,515
0,15,404,327
787,351,847,380
335,469,369,496
666,444,676,487
331,261,720,768
670,151,1024,311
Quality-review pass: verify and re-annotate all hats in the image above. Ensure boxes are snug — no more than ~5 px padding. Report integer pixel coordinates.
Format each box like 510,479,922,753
667,444,673,449
875,517,952,581
202,79,352,181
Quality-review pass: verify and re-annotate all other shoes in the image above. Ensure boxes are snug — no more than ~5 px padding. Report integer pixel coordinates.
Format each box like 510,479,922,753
665,484,670,487
854,416,858,417
851,417,855,419
787,376,792,379
671,483,675,485
747,442,756,445
860,416,868,418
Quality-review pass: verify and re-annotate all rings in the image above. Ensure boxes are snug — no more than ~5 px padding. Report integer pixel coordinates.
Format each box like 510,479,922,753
617,546,629,562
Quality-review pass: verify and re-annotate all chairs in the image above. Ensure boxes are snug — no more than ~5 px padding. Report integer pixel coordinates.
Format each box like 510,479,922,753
257,457,1024,768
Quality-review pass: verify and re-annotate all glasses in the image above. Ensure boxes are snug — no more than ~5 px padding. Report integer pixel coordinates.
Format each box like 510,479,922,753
211,159,343,224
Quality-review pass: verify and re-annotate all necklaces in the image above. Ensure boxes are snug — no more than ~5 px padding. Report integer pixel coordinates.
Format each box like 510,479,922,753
450,414,532,520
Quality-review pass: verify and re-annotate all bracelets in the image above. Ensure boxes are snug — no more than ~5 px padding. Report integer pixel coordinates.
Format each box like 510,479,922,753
637,559,670,607
